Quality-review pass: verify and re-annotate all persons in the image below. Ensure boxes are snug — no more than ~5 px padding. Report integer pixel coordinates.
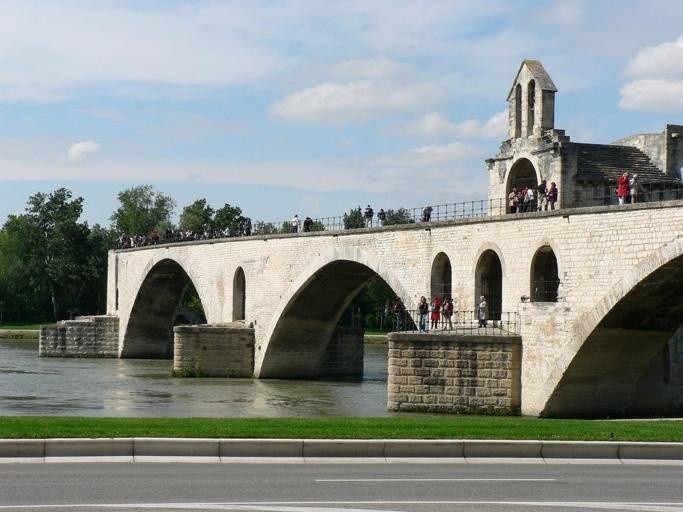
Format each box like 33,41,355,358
509,179,558,213
616,173,639,204
422,205,434,222
364,205,386,228
393,295,488,332
116,221,252,250
292,214,313,232
